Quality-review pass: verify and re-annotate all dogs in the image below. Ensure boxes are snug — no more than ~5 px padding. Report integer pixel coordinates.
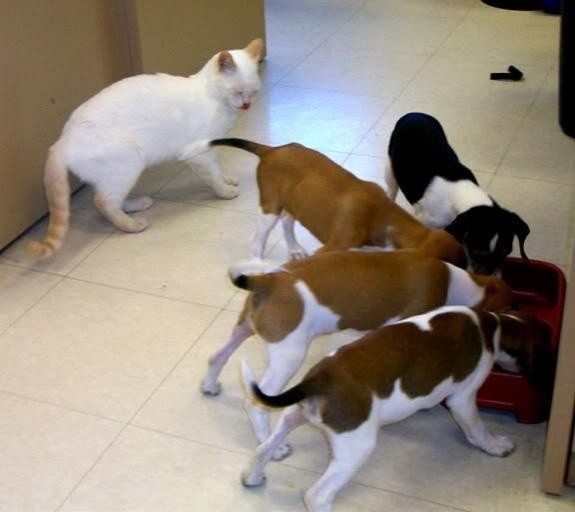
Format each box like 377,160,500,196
173,136,474,276
200,247,512,461
240,303,545,512
382,111,531,276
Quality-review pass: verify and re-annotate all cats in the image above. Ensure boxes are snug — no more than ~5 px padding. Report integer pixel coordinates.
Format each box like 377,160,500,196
25,37,265,262
